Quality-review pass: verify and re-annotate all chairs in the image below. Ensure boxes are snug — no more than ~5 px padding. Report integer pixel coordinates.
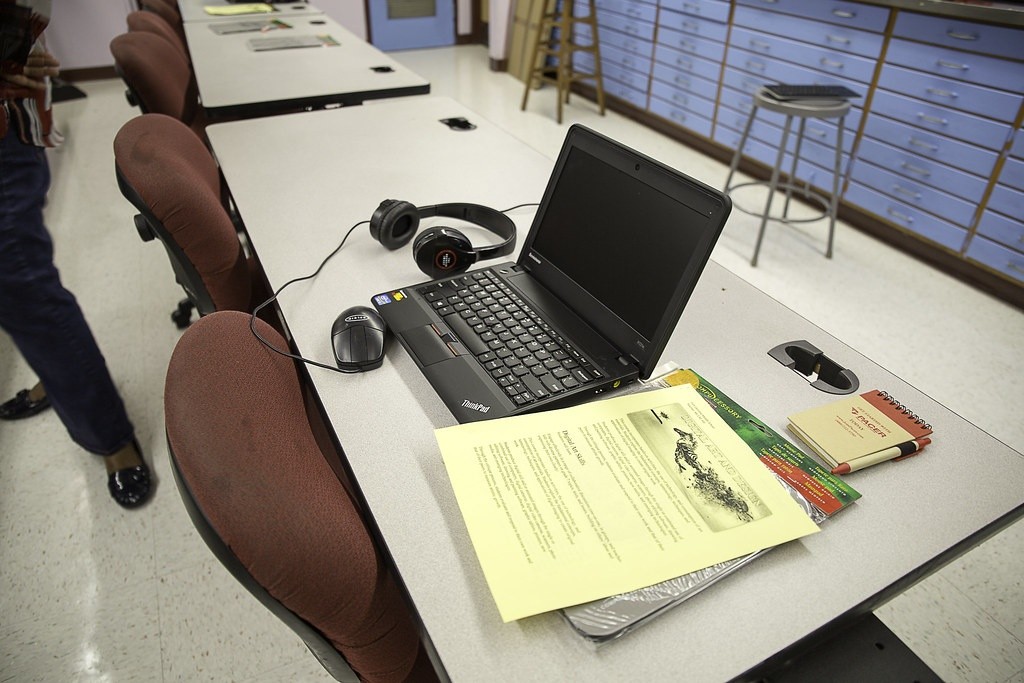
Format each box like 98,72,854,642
112,113,289,343
109,0,214,144
165,311,442,682
758,609,946,683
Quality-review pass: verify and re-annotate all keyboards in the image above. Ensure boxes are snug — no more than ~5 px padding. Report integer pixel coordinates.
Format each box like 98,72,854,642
761,85,862,99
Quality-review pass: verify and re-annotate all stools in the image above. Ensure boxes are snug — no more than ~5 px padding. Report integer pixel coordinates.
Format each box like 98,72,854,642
723,87,852,266
522,0,605,124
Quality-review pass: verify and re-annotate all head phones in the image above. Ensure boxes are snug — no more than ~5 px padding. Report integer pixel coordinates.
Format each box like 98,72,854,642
370,199,517,280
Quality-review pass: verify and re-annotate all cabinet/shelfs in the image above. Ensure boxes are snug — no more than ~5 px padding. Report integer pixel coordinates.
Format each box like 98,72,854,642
547,0,1023,312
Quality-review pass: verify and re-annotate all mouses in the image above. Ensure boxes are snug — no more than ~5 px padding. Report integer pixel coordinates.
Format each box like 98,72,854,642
331,305,388,371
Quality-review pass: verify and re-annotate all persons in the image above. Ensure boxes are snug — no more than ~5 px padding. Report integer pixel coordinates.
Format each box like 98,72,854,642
0,0,151,508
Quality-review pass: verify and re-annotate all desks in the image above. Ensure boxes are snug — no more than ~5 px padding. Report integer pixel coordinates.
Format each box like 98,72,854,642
177,0,431,123
204,93,1024,682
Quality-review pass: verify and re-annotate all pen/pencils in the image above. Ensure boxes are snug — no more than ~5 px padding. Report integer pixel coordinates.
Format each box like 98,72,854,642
831,436,931,477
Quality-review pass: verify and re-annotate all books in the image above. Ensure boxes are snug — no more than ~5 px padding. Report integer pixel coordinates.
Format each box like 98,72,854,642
787,390,934,468
557,369,863,655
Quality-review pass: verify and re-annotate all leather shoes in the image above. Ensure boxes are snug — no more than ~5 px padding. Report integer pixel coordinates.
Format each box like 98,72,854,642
105,433,151,510
0,389,52,420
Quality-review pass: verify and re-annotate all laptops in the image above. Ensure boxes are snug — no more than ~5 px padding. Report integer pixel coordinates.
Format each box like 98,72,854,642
370,124,733,429
